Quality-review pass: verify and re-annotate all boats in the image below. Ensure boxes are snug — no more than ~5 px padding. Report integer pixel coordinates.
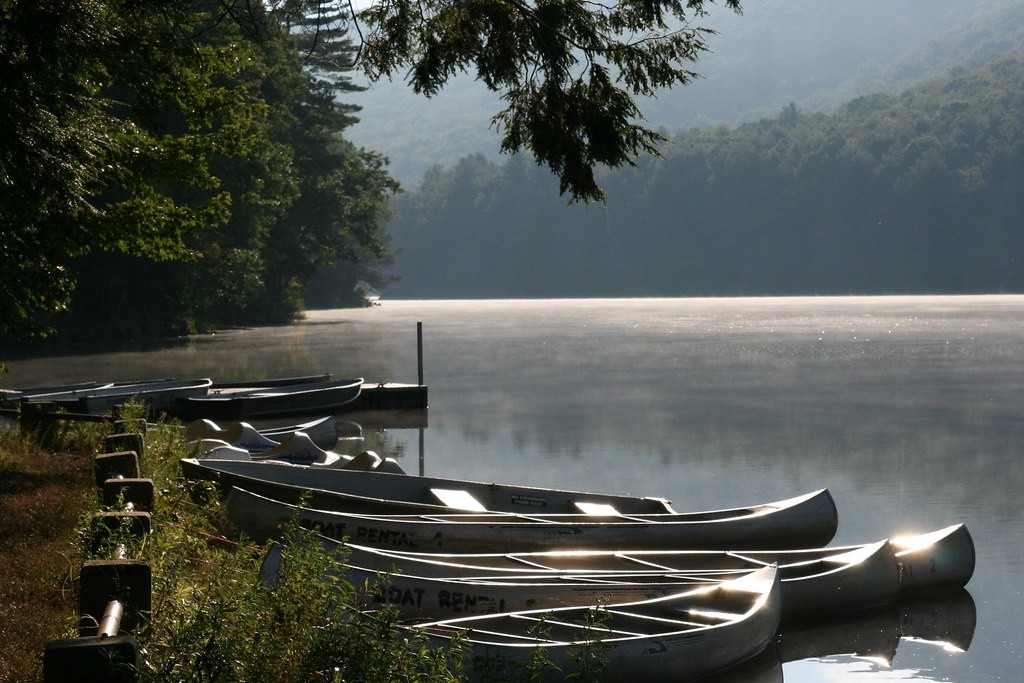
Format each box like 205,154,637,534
0,369,974,616
706,645,784,681
773,589,977,667
389,561,783,683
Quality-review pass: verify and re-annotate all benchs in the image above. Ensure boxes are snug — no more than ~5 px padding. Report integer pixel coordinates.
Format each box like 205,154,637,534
679,607,743,621
430,486,487,511
574,501,624,517
